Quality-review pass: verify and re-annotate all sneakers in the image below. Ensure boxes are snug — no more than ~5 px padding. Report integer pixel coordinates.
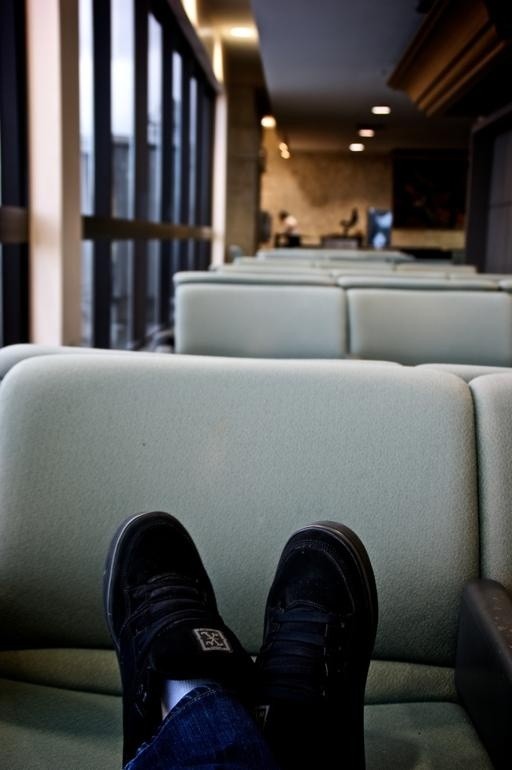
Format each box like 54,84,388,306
101,510,253,770
248,520,379,770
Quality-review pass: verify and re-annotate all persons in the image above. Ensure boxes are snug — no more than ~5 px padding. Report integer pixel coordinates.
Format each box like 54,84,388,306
101,509,378,770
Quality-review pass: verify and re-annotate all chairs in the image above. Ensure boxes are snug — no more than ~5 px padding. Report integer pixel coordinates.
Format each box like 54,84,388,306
0,246,512,770
